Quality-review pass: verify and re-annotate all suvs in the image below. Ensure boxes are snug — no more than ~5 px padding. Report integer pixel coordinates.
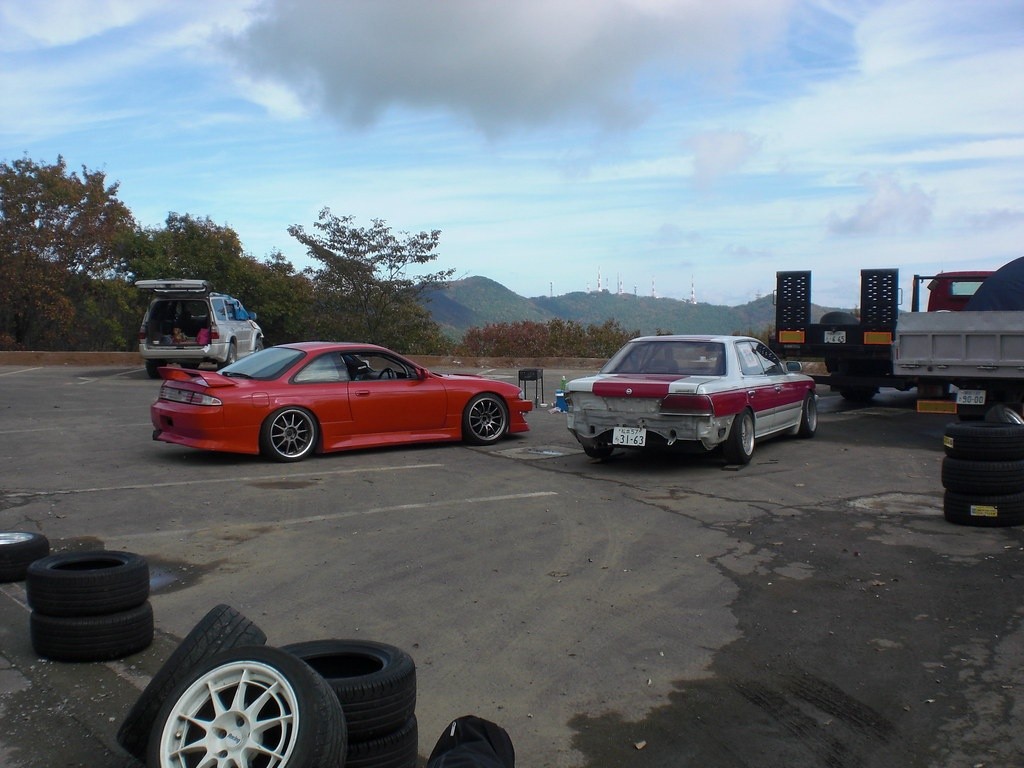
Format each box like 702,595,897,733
135,279,264,379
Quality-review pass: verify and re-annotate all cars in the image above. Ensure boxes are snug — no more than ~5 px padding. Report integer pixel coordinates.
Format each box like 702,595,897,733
564,333,817,464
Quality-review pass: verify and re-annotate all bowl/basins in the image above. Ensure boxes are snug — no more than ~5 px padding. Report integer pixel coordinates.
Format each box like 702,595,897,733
540,403,548,408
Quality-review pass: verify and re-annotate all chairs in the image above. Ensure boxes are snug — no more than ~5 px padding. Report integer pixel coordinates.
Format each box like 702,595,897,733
348,364,358,380
646,358,678,375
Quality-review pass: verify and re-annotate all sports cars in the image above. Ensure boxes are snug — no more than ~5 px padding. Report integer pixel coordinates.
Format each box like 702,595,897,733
150,340,533,463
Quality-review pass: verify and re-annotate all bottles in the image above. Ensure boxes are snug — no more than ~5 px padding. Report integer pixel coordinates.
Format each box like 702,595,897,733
561,376,567,390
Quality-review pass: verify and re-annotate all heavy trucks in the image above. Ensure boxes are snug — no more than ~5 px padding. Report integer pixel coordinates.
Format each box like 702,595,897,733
894,310,1024,424
767,268,997,402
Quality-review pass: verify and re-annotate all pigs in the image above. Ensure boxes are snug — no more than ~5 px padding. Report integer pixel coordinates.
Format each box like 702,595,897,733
173,327,189,342
